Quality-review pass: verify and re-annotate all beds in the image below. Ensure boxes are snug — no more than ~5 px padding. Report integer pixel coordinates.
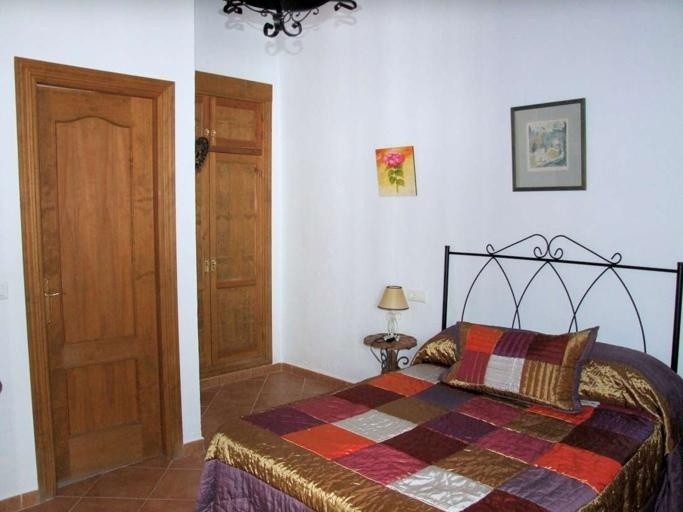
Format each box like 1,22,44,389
194,234,683,512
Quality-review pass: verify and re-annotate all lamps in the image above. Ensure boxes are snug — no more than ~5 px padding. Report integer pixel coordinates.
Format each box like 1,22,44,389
223,0,356,37
377,285,410,340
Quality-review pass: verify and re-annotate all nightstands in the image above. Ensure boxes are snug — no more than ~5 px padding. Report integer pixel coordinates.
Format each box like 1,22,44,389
363,333,417,374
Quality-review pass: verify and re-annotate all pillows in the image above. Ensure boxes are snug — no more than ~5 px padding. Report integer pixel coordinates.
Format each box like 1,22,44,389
411,321,682,415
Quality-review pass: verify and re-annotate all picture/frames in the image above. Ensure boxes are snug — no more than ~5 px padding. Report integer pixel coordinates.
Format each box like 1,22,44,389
510,97,586,193
